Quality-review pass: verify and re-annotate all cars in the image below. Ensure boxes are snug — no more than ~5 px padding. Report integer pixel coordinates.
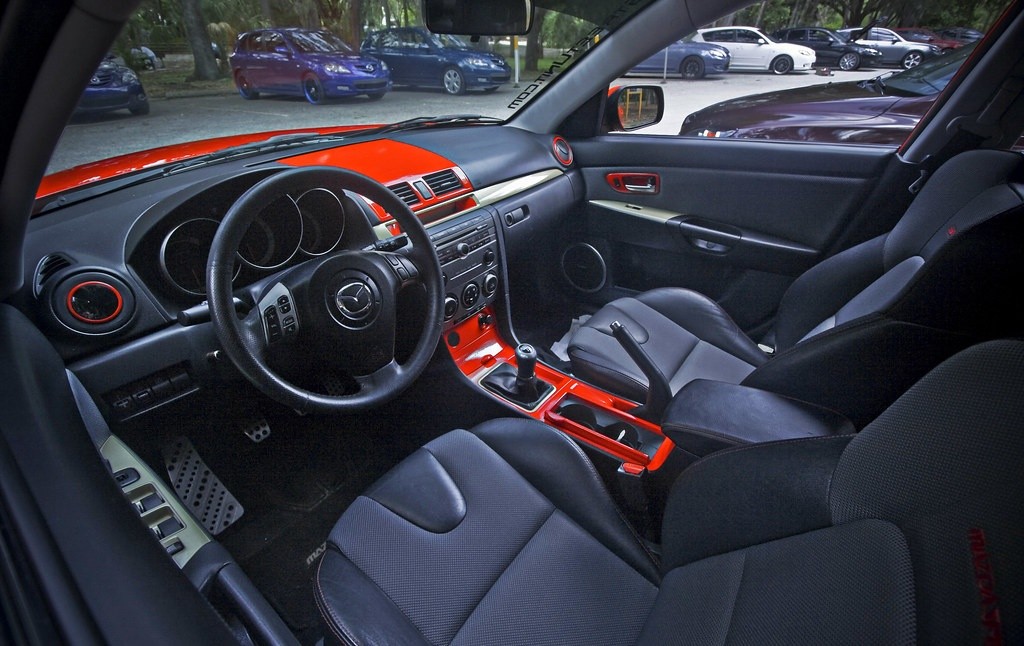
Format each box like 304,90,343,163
690,26,817,75
939,28,985,45
66,59,150,125
833,27,941,70
767,26,883,72
677,41,985,146
897,27,966,56
626,37,730,80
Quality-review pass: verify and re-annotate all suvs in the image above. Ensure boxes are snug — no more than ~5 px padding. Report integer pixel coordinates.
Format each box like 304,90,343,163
228,26,390,105
359,26,513,95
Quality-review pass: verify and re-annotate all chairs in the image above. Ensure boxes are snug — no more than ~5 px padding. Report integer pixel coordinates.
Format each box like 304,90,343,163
568,147,1024,417
306,341,1024,646
